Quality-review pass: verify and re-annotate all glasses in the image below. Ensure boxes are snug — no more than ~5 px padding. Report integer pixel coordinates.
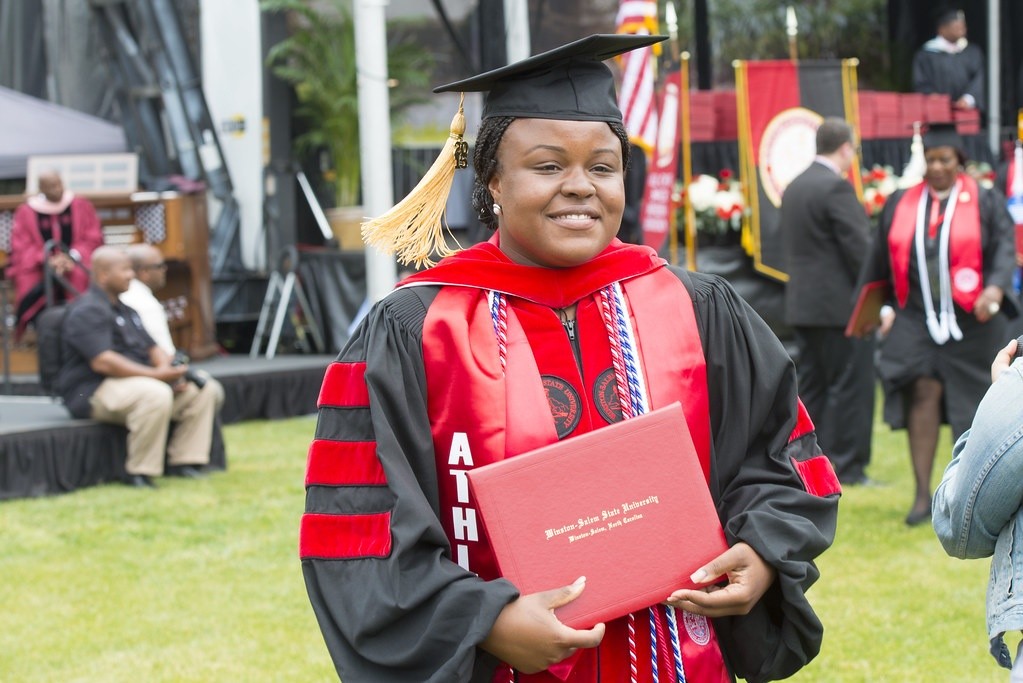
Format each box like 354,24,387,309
848,142,862,154
140,261,165,269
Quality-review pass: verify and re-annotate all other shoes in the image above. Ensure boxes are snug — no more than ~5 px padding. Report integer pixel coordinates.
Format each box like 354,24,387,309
124,474,156,490
168,465,205,480
905,497,932,525
837,473,885,488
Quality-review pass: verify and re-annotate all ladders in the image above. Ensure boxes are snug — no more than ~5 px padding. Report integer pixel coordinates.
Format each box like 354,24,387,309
249,247,325,358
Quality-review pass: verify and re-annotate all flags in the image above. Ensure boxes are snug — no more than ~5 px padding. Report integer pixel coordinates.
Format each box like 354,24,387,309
616,1,660,223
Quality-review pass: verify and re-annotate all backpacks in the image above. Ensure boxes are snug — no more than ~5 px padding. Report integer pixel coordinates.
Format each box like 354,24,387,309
36,303,74,398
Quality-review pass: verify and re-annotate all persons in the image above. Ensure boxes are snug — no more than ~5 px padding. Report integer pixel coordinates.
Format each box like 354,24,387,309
12,176,225,488
931,340,1023,683
299,31,843,683
780,120,1022,527
914,14,985,111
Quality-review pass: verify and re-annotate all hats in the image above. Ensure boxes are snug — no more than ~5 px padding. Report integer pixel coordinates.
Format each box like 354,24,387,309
361,34,670,271
898,120,974,185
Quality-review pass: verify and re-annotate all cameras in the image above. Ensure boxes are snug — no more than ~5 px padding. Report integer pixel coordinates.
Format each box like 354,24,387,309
170,350,208,389
